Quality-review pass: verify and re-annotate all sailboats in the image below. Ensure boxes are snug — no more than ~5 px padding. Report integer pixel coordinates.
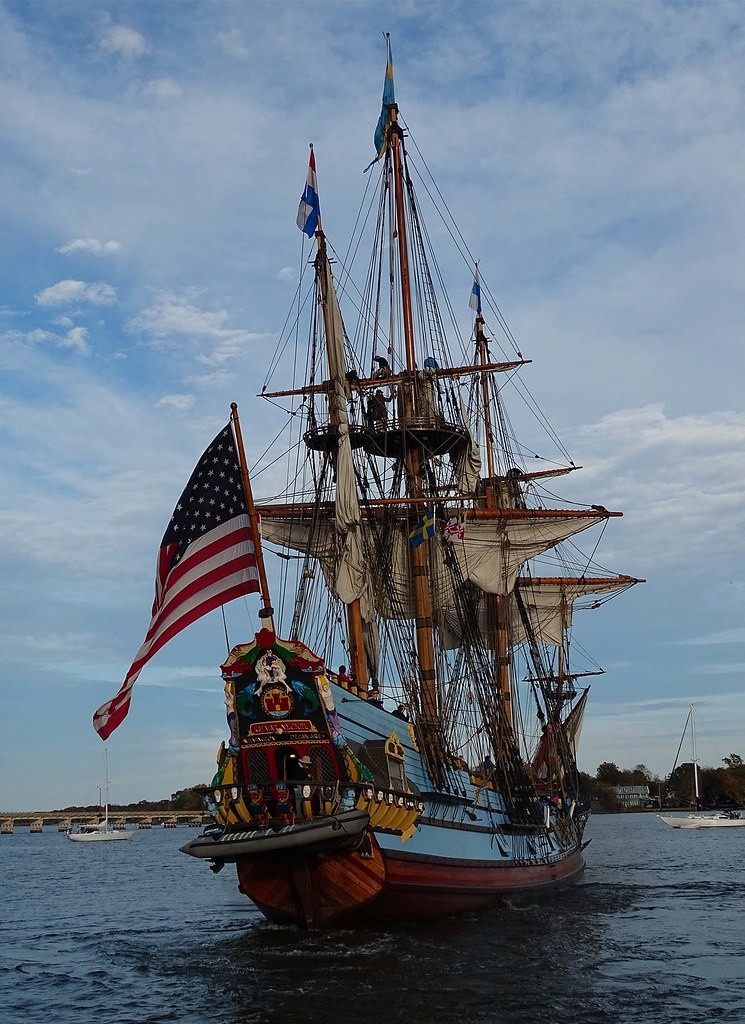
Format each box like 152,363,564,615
68,748,135,841
657,704,745,828
179,32,649,931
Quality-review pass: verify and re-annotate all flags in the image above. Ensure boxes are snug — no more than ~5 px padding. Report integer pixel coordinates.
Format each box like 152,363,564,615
94,424,261,741
441,510,467,540
297,151,320,239
409,508,435,548
362,42,394,173
468,268,481,313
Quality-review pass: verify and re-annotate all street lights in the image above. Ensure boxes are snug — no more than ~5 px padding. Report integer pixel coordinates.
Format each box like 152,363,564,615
97,786,101,815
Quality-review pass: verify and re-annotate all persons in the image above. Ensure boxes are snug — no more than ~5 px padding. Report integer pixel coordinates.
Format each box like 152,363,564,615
553,794,559,806
459,755,464,761
484,756,495,769
291,756,311,789
424,357,444,393
374,390,393,420
344,370,360,403
391,705,408,720
368,689,379,699
373,355,391,376
337,665,357,692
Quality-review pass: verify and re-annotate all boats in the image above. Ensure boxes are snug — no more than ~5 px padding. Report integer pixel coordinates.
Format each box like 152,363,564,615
63,823,86,838
